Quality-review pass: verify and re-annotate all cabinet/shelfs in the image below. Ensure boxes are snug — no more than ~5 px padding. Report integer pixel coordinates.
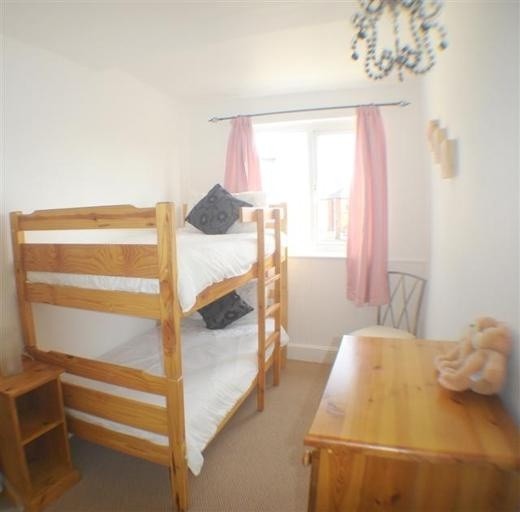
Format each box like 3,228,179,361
0,357,81,512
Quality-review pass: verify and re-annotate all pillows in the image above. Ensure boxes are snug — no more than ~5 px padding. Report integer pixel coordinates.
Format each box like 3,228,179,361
199,290,254,332
189,192,268,233
202,277,268,327
184,182,251,234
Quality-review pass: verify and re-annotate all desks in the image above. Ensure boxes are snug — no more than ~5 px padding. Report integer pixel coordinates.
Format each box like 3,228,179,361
303,334,520,512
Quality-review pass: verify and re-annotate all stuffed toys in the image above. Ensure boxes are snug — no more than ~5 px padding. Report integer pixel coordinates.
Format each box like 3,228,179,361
435,312,510,395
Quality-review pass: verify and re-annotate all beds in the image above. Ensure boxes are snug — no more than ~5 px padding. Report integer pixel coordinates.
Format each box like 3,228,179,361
8,204,287,512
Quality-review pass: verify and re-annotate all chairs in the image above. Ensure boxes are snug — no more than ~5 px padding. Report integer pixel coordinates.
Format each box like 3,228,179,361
348,271,427,338
